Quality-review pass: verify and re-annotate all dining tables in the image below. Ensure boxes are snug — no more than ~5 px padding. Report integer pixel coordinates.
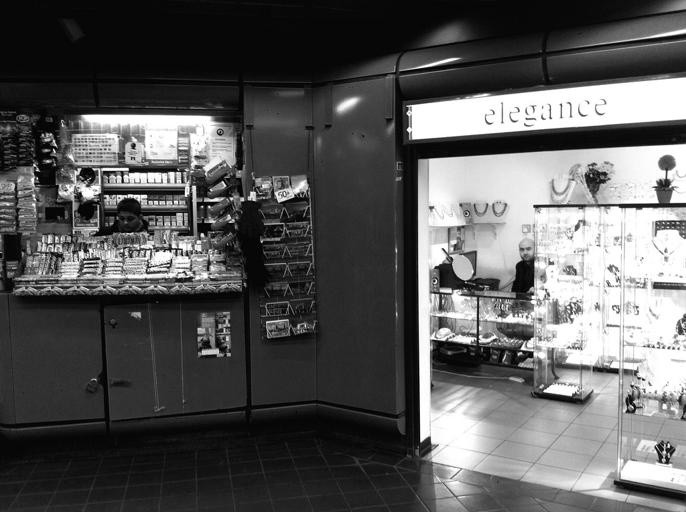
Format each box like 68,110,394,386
652,155,678,204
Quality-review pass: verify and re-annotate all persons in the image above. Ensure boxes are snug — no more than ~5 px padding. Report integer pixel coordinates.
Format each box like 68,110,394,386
95,197,154,237
510,238,535,293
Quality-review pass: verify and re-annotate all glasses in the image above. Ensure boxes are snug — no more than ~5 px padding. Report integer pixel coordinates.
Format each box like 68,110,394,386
428,199,467,263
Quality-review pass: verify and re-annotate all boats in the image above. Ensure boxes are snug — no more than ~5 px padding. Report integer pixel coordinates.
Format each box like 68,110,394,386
429,268,440,293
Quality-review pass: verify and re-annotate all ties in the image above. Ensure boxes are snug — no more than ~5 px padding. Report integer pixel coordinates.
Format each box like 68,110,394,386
456,249,481,277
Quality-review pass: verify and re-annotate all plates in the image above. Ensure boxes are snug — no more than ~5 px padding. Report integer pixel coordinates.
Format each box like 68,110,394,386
101,162,197,237
429,286,535,381
2,293,248,439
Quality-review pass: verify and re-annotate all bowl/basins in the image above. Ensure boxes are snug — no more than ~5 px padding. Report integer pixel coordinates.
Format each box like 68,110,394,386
651,239,683,262
552,178,570,195
434,208,445,219
649,306,667,321
660,443,671,449
443,208,453,217
656,446,675,458
492,204,507,217
473,204,489,217
